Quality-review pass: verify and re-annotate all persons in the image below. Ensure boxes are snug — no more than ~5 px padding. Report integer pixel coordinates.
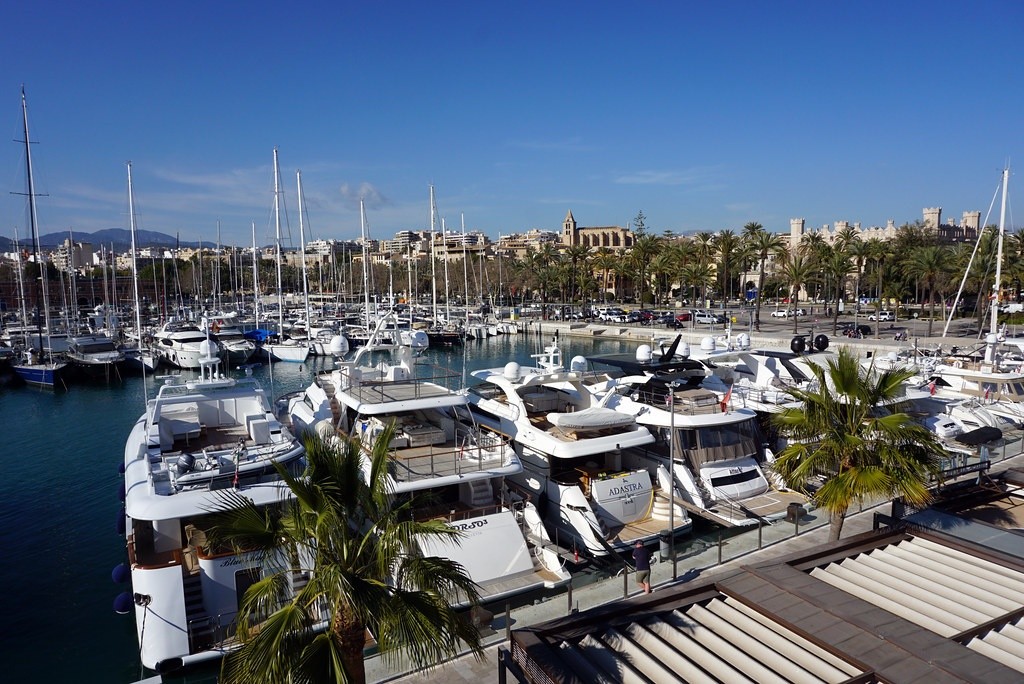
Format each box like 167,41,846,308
847,328,862,339
894,331,907,341
631,539,651,594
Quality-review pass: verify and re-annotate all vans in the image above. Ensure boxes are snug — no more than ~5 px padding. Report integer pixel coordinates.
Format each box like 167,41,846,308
696,313,719,324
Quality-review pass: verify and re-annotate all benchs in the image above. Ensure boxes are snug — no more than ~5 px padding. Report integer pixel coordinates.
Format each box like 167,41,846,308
918,318,938,322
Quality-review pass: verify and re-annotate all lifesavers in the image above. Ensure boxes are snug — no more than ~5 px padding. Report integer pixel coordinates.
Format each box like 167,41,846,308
128,534,135,565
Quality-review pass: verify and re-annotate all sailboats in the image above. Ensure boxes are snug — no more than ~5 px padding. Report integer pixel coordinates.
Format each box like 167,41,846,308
0,77,1023,676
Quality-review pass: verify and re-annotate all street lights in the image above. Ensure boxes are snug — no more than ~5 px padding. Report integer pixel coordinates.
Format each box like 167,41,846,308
665,380,682,580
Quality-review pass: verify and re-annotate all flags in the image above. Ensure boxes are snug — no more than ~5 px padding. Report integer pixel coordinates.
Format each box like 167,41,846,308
720,384,733,413
984,389,988,399
928,379,936,395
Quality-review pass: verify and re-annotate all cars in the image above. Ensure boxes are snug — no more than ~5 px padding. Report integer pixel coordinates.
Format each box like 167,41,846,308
790,309,807,316
657,315,675,324
771,309,792,318
850,325,872,335
678,313,692,321
717,315,730,323
867,310,895,322
